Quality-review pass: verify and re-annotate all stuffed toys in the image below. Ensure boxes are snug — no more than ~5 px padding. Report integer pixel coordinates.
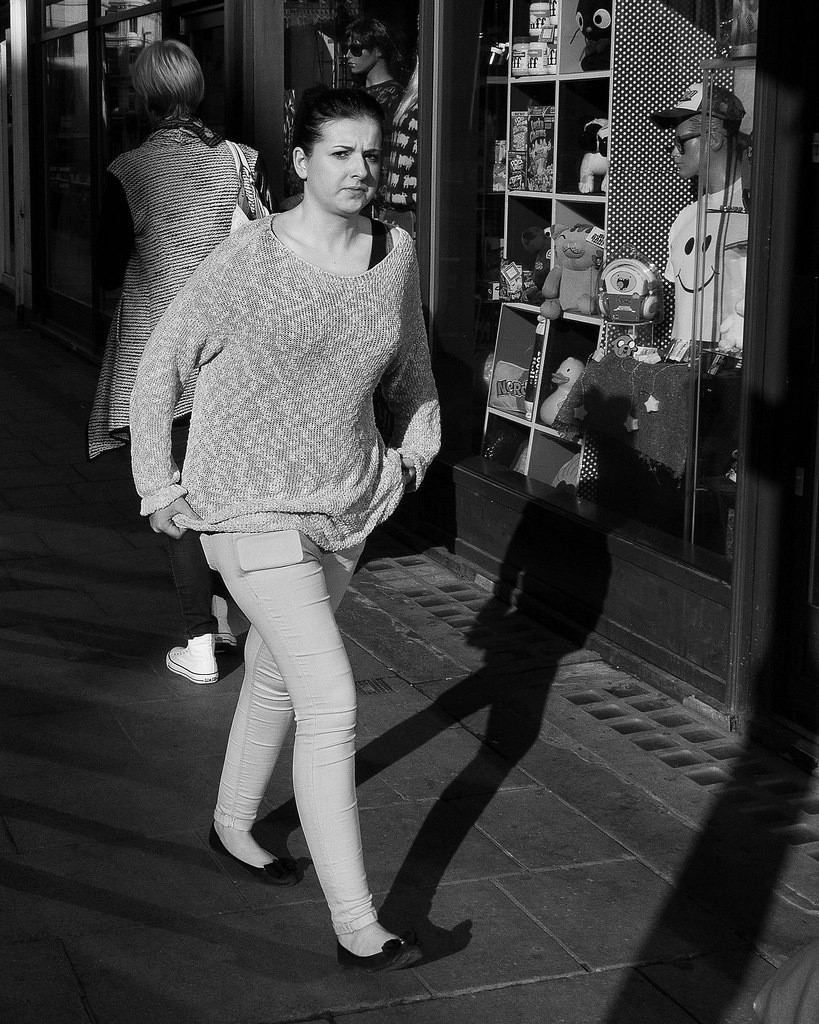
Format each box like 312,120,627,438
540,224,607,320
579,119,609,195
570,1,613,71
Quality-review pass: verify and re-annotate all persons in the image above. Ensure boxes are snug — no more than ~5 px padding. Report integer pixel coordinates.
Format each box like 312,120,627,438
88,39,278,684
646,83,751,360
346,18,403,108
130,88,443,977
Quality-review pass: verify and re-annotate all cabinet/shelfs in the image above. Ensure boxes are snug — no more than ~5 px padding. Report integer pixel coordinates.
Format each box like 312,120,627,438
429,0,759,733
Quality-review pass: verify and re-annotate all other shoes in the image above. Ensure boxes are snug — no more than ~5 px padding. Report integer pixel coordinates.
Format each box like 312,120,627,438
336,931,423,972
210,820,298,888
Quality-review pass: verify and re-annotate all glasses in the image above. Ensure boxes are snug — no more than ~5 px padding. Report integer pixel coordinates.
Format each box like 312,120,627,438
341,43,369,57
671,129,728,155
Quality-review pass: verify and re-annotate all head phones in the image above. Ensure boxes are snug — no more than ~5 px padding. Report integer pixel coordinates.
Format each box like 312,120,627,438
598,258,658,319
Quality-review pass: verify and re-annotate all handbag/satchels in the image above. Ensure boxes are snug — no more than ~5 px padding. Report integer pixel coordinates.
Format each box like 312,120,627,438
224,141,269,235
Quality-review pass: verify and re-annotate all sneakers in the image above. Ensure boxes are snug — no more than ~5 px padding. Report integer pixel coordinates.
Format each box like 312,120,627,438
166,633,218,684
211,596,249,648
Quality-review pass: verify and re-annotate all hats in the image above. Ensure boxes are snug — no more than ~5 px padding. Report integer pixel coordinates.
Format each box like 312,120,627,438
650,83,744,129
